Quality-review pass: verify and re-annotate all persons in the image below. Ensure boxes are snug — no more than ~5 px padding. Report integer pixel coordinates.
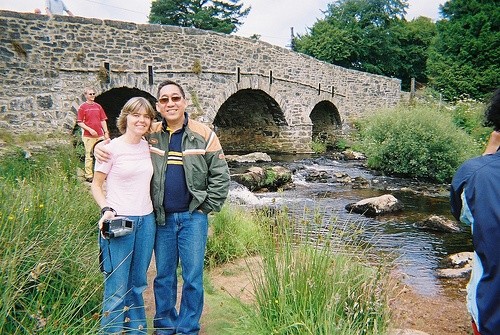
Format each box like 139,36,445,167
448,86,500,335
77,86,109,183
93,80,231,335
91,97,156,335
44,0,75,20
34,8,41,14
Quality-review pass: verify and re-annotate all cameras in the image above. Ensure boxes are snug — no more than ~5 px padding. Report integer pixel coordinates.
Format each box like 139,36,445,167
103,216,134,238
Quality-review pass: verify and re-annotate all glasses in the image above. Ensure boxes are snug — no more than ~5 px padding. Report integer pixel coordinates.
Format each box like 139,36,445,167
89,93,96,95
159,96,183,104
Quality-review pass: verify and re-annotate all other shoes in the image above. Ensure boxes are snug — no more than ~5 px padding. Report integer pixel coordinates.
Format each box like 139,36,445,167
86,178,93,182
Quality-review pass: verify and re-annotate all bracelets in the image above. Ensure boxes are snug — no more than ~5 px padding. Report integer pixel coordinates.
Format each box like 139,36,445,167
101,206,117,215
104,131,109,134
